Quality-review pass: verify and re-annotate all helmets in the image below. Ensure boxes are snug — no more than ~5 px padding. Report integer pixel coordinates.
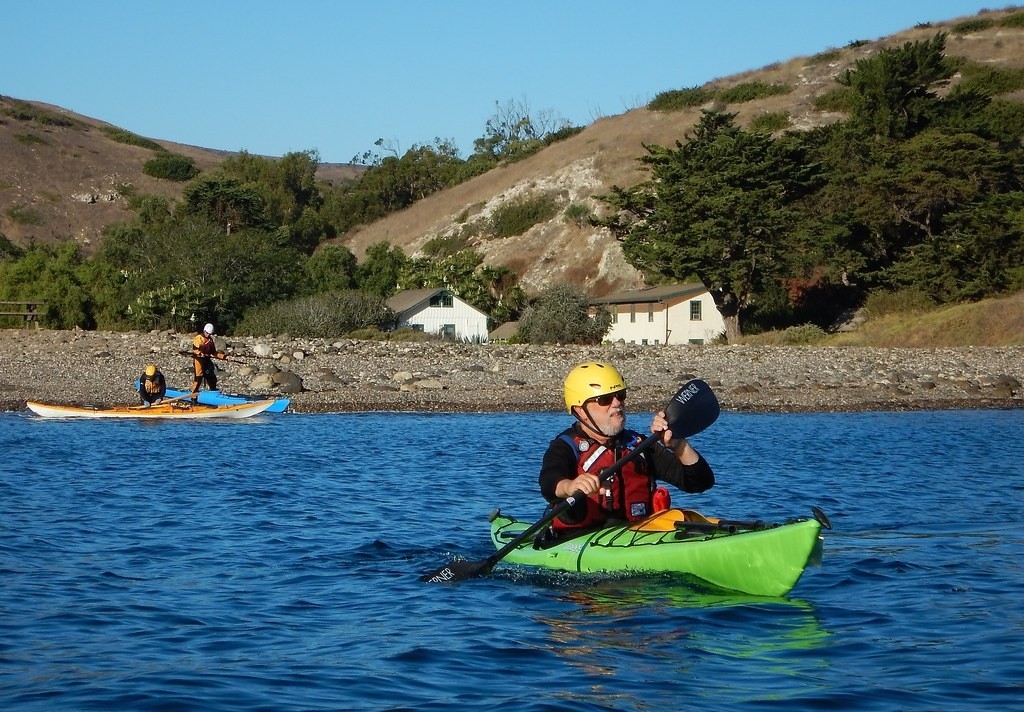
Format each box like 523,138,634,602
562,362,627,416
146,366,156,375
203,323,214,333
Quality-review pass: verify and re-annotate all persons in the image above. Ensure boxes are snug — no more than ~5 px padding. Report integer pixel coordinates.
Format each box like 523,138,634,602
539,361,715,535
191,323,225,405
140,366,166,408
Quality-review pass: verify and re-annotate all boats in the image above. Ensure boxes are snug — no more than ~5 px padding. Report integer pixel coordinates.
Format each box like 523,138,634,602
134,380,293,414
21,397,274,422
490,508,821,600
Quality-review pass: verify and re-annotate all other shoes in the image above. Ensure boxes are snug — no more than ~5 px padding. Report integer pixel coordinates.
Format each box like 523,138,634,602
191,400,201,406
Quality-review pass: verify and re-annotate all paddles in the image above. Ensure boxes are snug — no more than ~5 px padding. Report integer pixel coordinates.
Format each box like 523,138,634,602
178,350,249,365
416,379,721,585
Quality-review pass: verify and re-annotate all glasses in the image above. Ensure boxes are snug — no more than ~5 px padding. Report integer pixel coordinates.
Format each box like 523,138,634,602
206,332,211,335
587,389,626,406
